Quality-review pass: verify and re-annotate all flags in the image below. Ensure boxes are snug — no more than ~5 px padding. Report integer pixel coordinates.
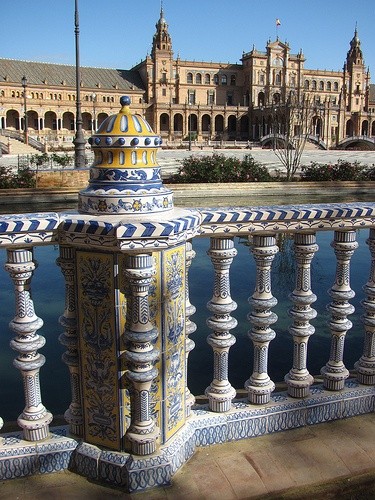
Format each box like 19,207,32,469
276,19,280,25
